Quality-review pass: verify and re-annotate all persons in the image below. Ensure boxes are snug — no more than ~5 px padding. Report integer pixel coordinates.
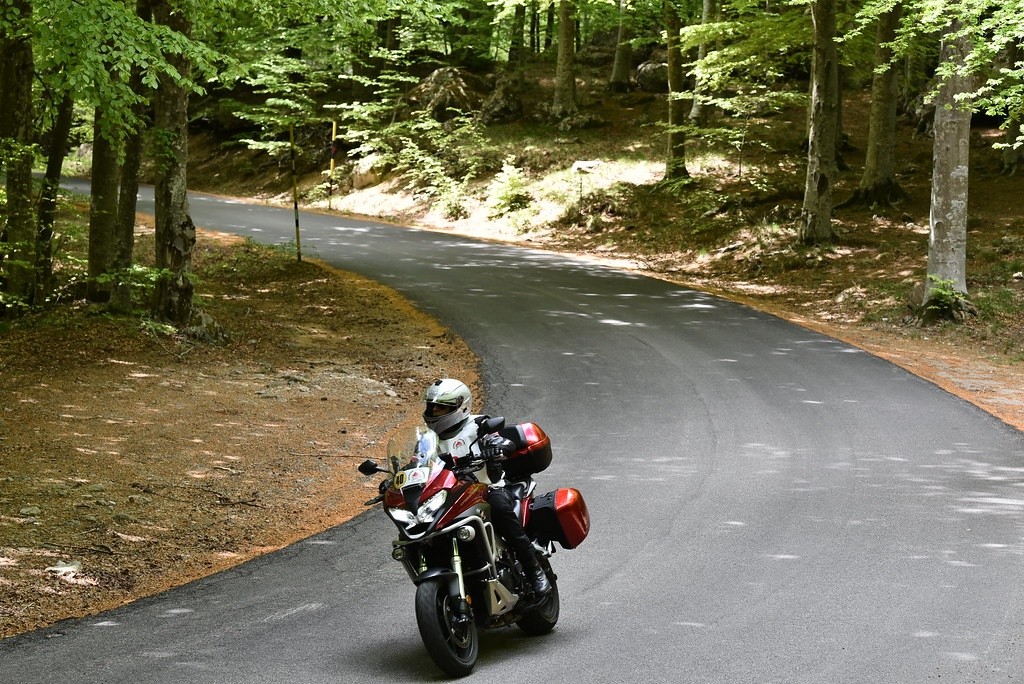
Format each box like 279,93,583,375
379,379,553,611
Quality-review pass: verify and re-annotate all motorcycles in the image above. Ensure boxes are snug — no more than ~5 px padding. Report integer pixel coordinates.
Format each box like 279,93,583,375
357,422,590,678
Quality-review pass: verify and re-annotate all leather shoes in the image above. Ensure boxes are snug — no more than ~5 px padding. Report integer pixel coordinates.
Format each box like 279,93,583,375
528,561,552,598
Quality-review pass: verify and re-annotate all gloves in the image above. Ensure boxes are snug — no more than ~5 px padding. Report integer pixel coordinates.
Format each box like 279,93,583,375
379,478,395,494
482,448,501,464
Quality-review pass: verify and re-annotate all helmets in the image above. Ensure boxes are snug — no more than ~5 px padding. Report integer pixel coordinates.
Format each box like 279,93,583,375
421,379,472,435
